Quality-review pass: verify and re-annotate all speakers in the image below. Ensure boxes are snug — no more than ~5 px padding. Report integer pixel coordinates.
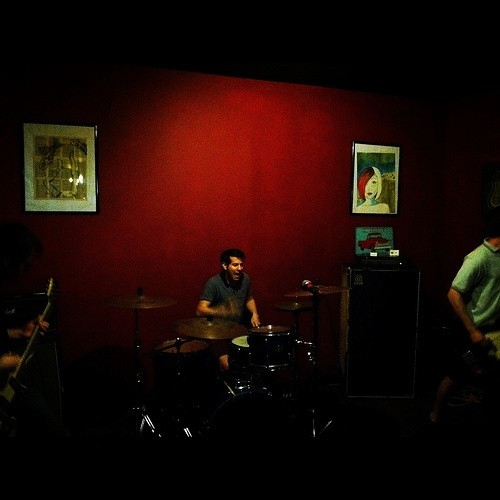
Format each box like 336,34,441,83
344,263,422,400
14,290,63,395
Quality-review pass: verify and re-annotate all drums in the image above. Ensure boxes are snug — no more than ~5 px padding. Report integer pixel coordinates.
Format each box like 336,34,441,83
245,325,294,369
229,334,255,366
460,330,500,372
202,377,292,439
150,341,211,394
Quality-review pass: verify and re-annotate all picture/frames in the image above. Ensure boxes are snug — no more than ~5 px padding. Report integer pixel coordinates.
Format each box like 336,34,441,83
349,138,403,220
352,225,395,258
23,121,99,216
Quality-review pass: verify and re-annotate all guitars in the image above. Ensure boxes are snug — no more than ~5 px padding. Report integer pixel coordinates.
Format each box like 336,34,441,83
0,278,55,404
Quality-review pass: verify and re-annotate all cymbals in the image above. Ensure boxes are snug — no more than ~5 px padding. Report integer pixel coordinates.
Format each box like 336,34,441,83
166,317,248,340
283,284,353,298
274,300,313,311
104,292,177,310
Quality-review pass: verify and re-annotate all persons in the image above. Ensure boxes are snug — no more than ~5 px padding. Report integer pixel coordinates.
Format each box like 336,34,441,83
0,220,50,411
196,249,262,375
429,187,500,423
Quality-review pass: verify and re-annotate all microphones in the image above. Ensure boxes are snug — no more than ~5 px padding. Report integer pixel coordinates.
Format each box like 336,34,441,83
302,279,319,295
